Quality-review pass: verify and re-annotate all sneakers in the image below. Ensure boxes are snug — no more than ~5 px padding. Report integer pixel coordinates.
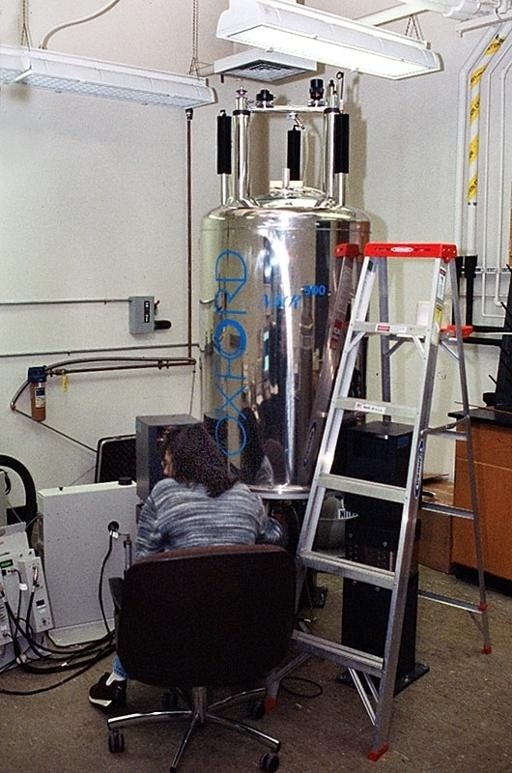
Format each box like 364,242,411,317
89,672,126,707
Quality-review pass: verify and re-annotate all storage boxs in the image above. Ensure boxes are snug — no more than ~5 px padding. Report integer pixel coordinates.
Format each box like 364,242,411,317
417,472,455,574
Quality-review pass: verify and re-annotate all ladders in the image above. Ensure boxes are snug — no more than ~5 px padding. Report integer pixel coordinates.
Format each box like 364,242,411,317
296,243,364,486
262,241,493,762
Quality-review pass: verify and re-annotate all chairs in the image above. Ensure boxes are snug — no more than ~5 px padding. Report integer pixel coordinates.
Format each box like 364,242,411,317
93,433,138,482
108,543,298,773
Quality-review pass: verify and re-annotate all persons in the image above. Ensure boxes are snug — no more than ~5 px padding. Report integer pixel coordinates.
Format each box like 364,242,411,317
234,406,275,486
86,420,284,720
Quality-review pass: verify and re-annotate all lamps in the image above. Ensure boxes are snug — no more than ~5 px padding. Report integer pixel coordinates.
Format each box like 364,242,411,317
215,0,443,79
0,0,217,110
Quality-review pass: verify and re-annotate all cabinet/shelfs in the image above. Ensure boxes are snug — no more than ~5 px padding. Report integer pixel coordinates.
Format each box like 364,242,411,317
453,419,512,595
